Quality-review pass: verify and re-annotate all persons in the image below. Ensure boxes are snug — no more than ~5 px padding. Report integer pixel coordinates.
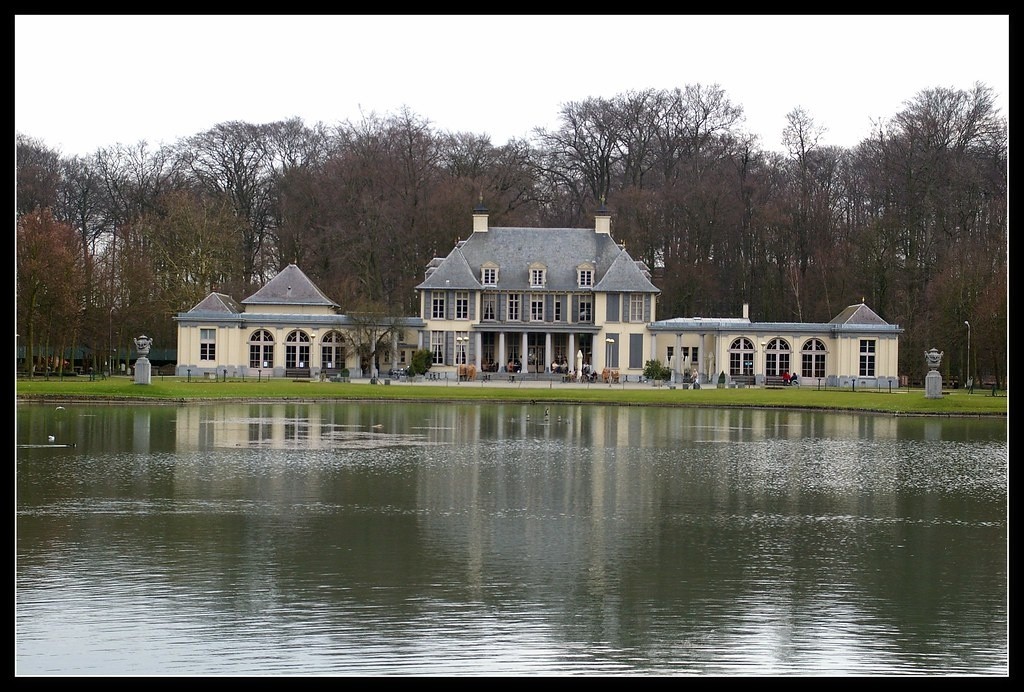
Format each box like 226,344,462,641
782,371,790,386
562,360,568,372
583,361,590,370
690,368,699,383
552,361,558,373
791,373,797,385
967,376,974,394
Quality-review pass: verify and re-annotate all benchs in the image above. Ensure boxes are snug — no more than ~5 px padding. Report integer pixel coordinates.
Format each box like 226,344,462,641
285,370,310,379
766,375,791,387
731,375,756,386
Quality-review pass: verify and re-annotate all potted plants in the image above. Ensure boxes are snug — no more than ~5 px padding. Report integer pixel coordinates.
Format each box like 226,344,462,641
341,368,350,377
717,371,725,388
642,360,661,384
652,359,670,387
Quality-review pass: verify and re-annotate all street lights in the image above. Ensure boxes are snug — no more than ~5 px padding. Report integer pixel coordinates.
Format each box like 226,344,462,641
964,319,971,390
109,307,116,374
456,336,470,364
604,338,616,387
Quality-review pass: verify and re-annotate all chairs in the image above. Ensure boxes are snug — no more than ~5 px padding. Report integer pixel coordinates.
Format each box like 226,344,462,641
601,369,611,383
611,371,620,383
